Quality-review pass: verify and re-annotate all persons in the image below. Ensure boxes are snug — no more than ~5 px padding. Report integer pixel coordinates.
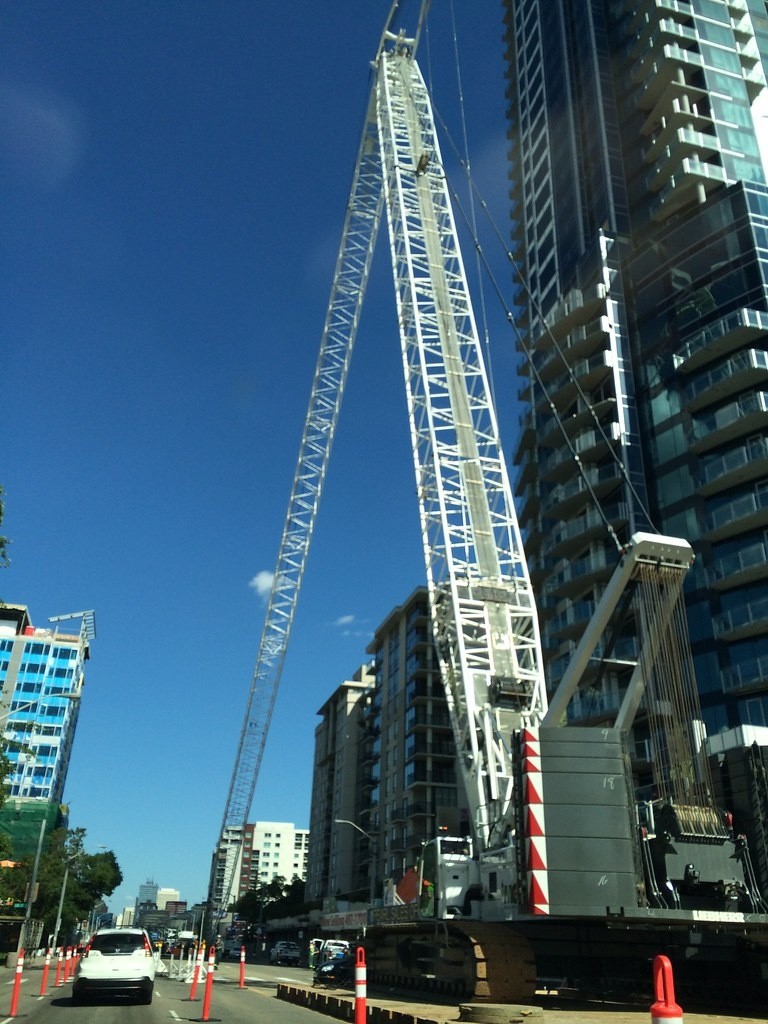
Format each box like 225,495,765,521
214,935,224,970
161,940,167,954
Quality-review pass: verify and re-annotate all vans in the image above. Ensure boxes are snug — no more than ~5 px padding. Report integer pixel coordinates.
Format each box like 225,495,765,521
307,937,350,969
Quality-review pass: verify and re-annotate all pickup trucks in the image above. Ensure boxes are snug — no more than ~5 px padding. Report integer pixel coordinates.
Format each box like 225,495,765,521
269,940,302,969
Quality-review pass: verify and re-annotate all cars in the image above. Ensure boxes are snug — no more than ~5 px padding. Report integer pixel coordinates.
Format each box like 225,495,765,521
229,947,242,959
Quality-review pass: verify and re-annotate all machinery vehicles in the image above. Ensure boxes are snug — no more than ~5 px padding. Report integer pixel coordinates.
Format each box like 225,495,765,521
204,0,765,1009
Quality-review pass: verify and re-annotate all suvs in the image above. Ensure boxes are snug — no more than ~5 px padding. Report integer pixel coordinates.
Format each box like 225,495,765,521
72,927,157,1005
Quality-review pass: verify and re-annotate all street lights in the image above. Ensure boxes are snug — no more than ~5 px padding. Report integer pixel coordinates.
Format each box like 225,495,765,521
334,818,377,903
51,844,108,960
91,899,112,938
